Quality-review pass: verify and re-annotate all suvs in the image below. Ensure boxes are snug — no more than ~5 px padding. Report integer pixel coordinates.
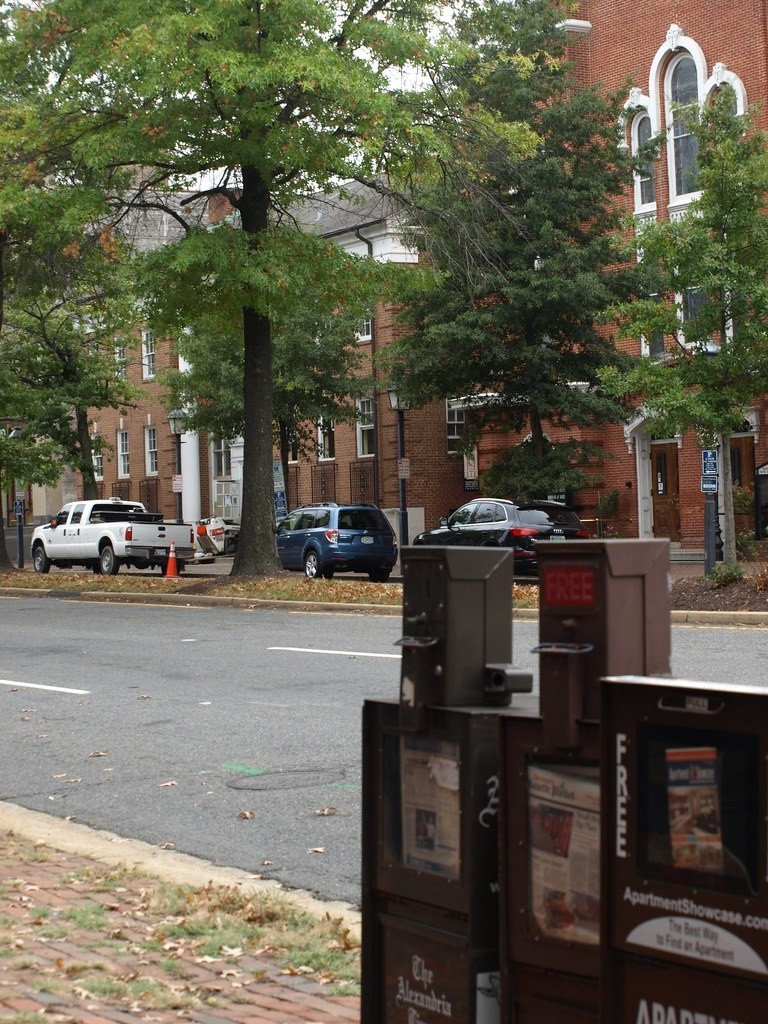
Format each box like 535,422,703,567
275,503,398,581
414,498,590,578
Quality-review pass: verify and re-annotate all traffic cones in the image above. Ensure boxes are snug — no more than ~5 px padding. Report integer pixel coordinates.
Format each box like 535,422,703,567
164,540,180,578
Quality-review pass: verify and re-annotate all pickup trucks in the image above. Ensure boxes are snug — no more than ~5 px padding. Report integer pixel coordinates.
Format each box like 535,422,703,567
30,497,197,575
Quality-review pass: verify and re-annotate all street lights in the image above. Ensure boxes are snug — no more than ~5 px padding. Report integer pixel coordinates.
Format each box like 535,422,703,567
167,406,189,523
690,338,721,578
9,427,24,568
387,382,410,573
